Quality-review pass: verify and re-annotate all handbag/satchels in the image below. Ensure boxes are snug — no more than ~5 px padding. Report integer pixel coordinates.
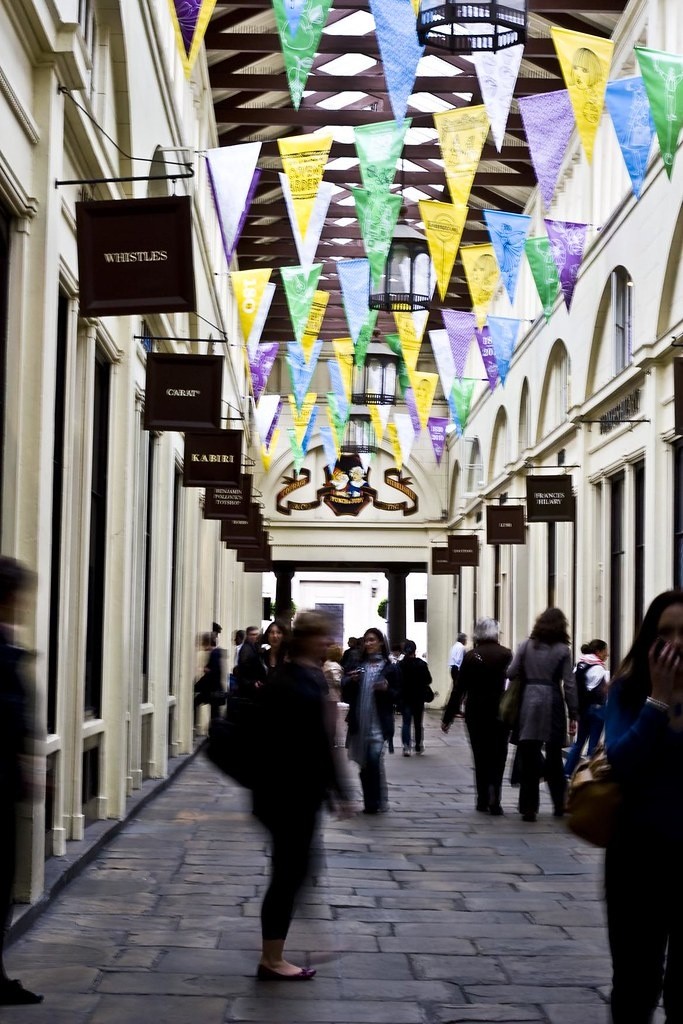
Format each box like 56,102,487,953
499,677,523,726
565,745,621,848
424,685,434,703
202,692,255,790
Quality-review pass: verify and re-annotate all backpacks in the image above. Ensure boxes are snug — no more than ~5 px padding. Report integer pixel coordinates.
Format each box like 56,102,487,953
570,660,606,726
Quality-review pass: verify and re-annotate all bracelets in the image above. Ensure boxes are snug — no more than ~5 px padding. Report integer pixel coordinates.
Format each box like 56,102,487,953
646,696,670,710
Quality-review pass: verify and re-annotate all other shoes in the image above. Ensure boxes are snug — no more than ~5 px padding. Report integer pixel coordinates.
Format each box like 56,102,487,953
553,806,565,818
403,750,411,756
258,965,317,981
0,988,45,1006
416,747,425,756
522,812,536,821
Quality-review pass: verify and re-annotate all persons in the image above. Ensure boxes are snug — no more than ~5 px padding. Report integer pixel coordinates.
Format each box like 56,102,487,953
563,639,611,772
242,611,353,979
0,553,46,1005
440,618,514,815
396,638,434,757
320,636,405,748
449,633,467,718
602,589,683,1024
340,628,403,813
504,608,578,822
194,621,287,737
